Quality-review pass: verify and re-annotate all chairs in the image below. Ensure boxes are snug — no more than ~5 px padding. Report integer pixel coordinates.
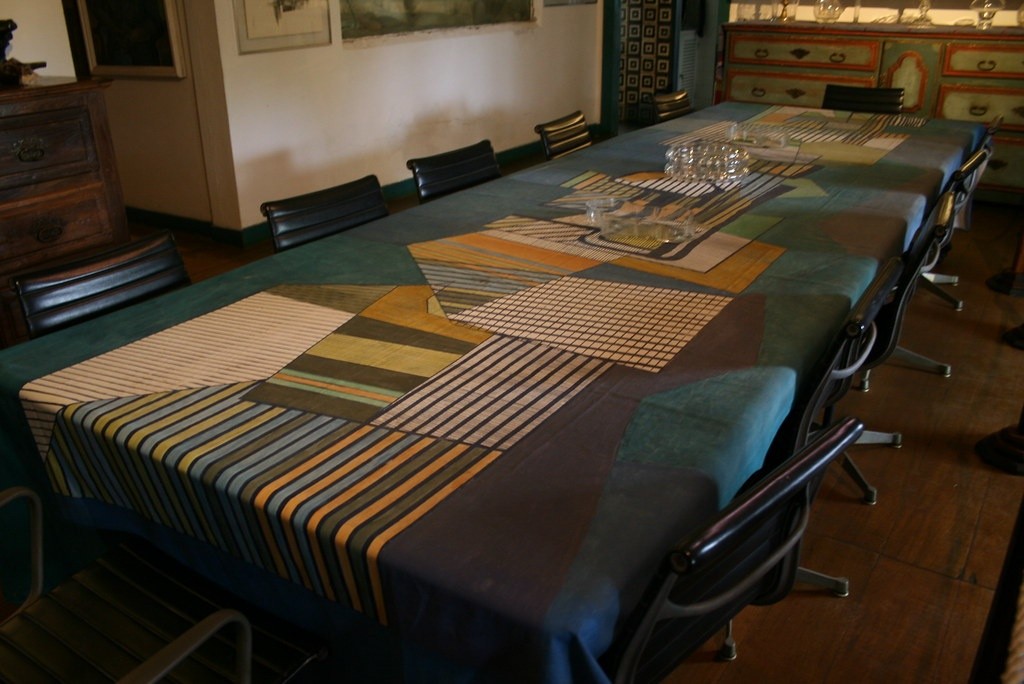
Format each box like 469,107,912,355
820,85,905,116
535,111,594,161
406,139,503,206
654,92,697,122
778,121,1005,505
1,98,988,684
610,418,863,683
13,230,195,340
259,173,390,255
0,488,333,684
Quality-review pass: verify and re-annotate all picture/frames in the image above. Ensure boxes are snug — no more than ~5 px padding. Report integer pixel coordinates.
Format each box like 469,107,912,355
330,0,546,52
232,1,331,55
76,0,187,78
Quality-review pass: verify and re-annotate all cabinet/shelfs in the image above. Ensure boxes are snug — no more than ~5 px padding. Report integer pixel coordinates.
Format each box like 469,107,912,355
714,24,1023,206
1,75,130,352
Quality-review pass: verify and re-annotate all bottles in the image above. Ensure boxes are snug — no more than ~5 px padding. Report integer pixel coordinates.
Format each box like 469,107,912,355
814,0,842,24
